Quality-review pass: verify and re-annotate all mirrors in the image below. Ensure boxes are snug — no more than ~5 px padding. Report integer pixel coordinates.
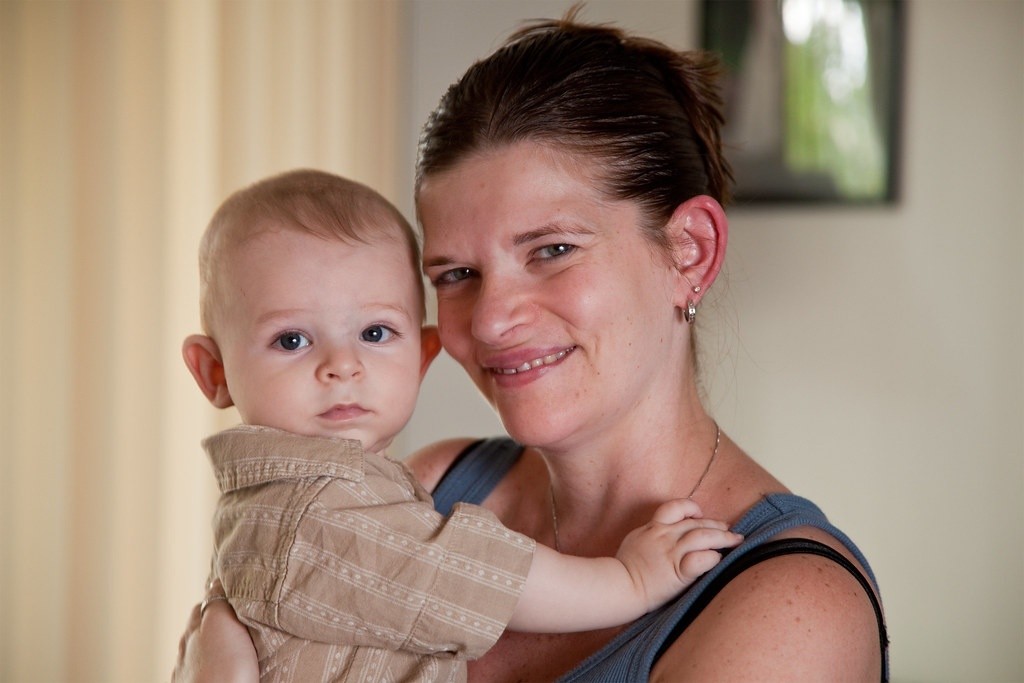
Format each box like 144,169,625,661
694,0,906,211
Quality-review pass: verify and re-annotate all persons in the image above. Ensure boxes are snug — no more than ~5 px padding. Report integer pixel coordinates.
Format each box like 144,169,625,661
172,18,890,683
181,169,745,683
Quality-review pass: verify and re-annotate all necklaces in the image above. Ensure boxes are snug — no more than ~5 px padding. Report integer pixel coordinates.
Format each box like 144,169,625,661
550,419,720,550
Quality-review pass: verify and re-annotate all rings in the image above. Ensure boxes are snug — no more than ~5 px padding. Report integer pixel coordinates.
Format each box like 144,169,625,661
201,593,225,617
